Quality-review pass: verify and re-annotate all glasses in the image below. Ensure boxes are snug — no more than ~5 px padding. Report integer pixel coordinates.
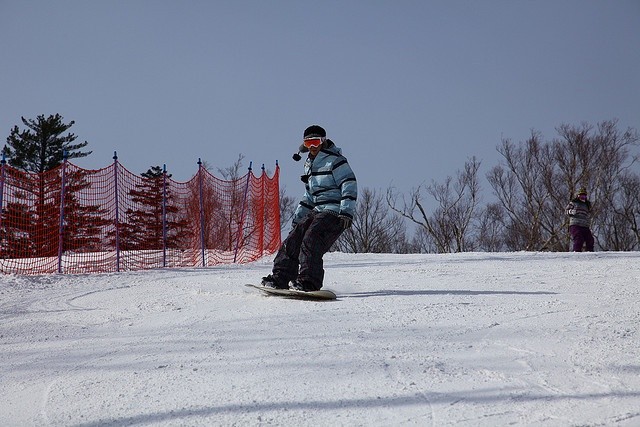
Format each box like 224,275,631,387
303,136,326,149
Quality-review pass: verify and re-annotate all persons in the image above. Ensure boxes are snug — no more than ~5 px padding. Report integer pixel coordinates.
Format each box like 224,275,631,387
566,187,596,252
261,126,357,293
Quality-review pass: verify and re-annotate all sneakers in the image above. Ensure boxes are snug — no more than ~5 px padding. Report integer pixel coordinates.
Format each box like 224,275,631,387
261,275,280,288
288,280,317,291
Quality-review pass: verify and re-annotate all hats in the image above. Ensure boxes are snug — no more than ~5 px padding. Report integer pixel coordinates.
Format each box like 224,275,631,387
577,188,587,194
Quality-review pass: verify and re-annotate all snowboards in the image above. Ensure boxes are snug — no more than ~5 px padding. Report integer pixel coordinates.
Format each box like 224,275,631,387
244,282,337,300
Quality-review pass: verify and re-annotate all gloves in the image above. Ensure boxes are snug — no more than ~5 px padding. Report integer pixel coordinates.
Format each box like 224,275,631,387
569,209,576,215
338,212,353,229
